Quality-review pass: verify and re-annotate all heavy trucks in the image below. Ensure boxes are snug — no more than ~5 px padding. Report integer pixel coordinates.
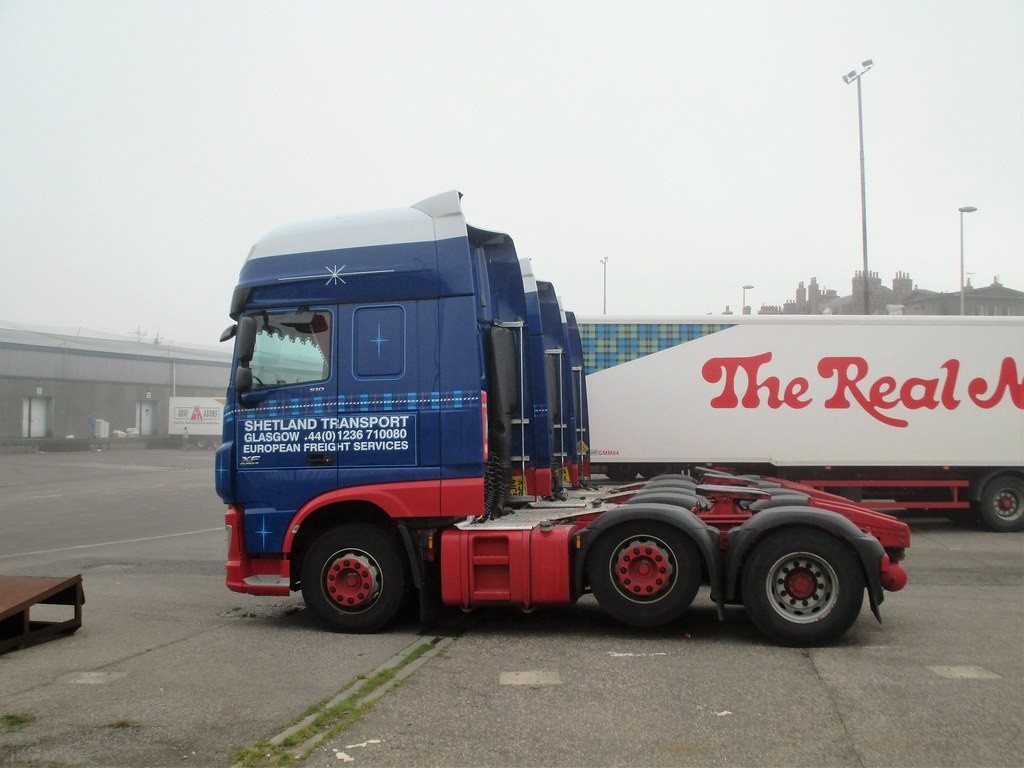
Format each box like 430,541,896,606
213,191,909,645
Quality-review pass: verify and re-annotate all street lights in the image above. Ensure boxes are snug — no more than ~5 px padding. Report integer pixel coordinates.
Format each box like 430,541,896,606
959,205,978,314
742,284,754,315
